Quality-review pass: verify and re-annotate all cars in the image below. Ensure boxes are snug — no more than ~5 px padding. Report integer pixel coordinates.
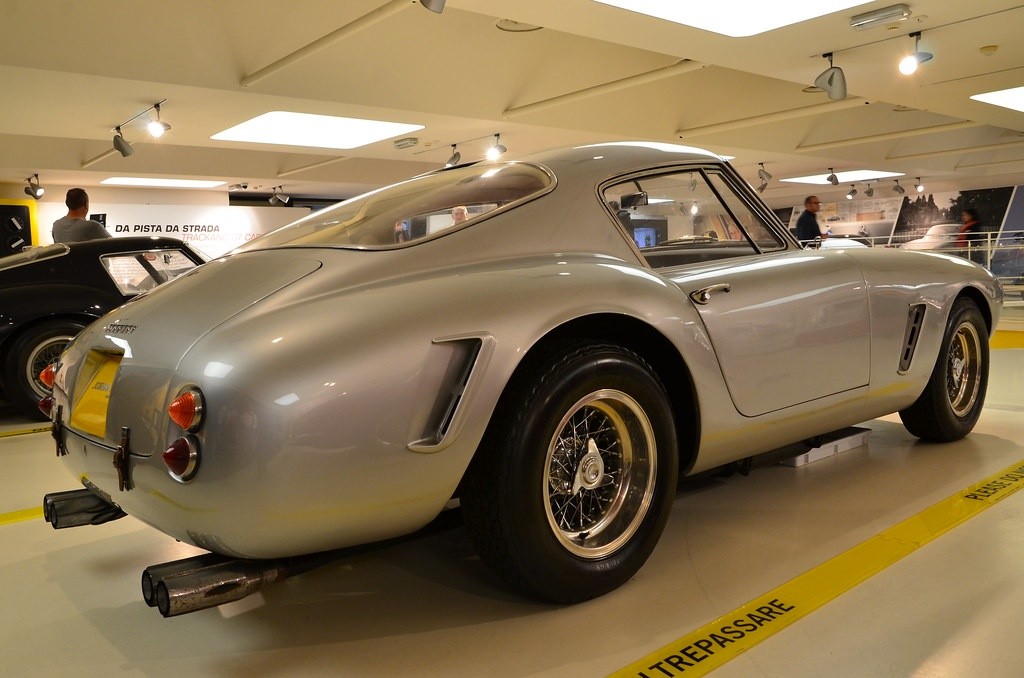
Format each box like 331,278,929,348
41,141,1007,623
2,236,210,424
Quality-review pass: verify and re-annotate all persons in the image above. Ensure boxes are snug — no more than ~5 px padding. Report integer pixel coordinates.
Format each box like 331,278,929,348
52,188,113,273
395,223,409,245
609,200,634,241
448,206,469,226
795,196,822,246
955,208,982,265
